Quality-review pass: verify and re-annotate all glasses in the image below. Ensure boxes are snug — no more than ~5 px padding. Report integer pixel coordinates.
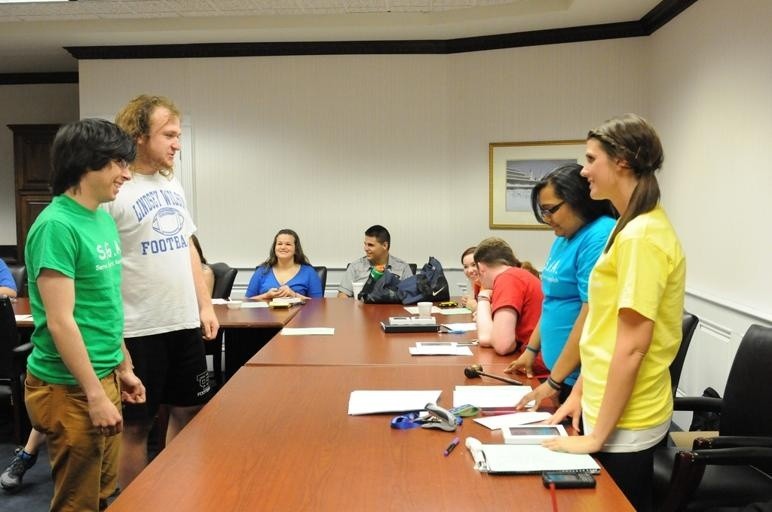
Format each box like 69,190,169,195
536,198,568,218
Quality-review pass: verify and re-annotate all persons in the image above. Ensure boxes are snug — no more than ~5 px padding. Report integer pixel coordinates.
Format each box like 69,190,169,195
0,426,49,494
101,92,221,493
190,232,215,297
21,117,149,512
337,223,415,301
541,110,688,512
460,246,479,321
502,161,622,437
472,236,554,379
1,256,17,299
245,228,324,299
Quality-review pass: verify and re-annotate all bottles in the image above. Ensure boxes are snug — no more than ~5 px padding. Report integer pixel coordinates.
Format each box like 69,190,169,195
369,265,386,280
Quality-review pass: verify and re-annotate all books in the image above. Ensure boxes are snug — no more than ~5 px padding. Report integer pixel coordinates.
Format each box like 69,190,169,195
379,320,441,333
480,442,602,476
451,383,536,415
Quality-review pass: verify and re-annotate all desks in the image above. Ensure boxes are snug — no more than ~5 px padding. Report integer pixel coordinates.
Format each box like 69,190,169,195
246,298,536,366
98,366,638,512
12,297,308,332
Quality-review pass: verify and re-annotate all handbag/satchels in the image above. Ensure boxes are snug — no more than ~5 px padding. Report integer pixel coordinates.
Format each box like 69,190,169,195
362,258,450,305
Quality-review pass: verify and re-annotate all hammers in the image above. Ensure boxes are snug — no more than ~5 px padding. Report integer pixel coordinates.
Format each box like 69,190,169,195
464,364,523,385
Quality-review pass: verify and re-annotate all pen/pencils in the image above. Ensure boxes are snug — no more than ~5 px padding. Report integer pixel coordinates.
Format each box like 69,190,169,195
438,330,464,334
479,410,516,416
444,436,459,456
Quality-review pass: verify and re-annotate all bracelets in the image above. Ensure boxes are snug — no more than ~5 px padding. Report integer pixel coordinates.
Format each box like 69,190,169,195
524,345,539,355
547,377,562,391
476,294,490,302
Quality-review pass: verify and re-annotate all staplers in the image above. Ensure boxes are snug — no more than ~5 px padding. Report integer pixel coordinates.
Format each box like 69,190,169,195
421,402,457,432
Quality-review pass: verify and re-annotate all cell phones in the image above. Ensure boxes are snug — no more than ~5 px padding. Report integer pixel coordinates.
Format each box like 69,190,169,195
438,301,458,307
542,472,595,489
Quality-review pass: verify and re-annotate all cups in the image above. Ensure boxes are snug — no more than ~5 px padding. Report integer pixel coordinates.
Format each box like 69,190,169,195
417,301,433,318
455,283,469,309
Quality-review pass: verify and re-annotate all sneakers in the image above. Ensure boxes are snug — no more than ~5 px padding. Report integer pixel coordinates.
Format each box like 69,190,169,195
1,446,40,491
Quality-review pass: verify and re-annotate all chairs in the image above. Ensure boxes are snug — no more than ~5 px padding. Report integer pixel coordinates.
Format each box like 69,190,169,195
208,263,239,388
5,263,28,295
407,263,417,276
309,266,327,297
671,309,702,392
0,297,41,446
642,320,772,511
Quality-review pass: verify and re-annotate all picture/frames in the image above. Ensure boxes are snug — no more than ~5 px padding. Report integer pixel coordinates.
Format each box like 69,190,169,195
487,139,595,233
